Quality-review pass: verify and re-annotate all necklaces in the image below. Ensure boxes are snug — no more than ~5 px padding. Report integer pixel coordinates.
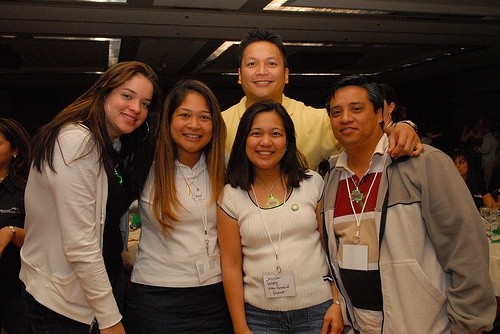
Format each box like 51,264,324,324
254,175,285,208
176,159,200,195
108,154,126,186
349,174,370,203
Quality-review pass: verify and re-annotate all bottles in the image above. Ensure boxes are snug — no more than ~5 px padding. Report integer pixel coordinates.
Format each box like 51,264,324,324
496,189,500,217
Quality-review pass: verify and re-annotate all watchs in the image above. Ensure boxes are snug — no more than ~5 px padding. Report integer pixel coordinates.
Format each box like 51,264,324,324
401,120,417,132
8,226,16,238
332,300,340,304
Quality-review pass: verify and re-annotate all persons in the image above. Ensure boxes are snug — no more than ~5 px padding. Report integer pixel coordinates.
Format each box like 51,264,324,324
217,99,344,334
112,80,236,334
379,84,500,210
19,61,159,334
0,118,33,334
221,26,423,173
321,75,497,334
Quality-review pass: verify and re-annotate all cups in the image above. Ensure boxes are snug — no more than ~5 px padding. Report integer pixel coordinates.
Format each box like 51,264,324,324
480,207,491,220
